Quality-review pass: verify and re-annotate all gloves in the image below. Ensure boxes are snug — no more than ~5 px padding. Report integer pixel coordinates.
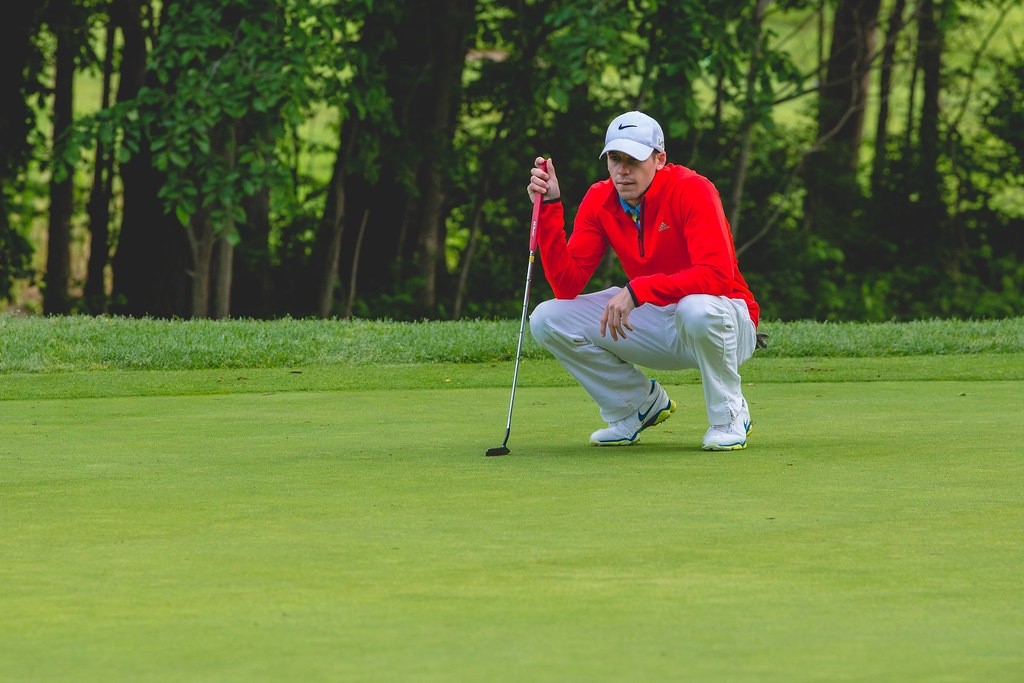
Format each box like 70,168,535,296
755,333,769,350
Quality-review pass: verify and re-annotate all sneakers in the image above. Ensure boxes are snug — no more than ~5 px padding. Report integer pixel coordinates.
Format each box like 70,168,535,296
590,378,676,445
702,397,754,450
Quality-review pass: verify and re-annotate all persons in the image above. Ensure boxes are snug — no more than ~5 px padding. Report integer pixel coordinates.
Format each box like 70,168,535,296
527,110,760,452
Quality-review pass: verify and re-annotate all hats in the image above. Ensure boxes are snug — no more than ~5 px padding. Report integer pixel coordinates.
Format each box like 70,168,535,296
599,111,665,161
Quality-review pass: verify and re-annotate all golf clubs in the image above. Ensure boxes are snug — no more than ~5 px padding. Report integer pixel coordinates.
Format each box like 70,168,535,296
485,158,549,457
